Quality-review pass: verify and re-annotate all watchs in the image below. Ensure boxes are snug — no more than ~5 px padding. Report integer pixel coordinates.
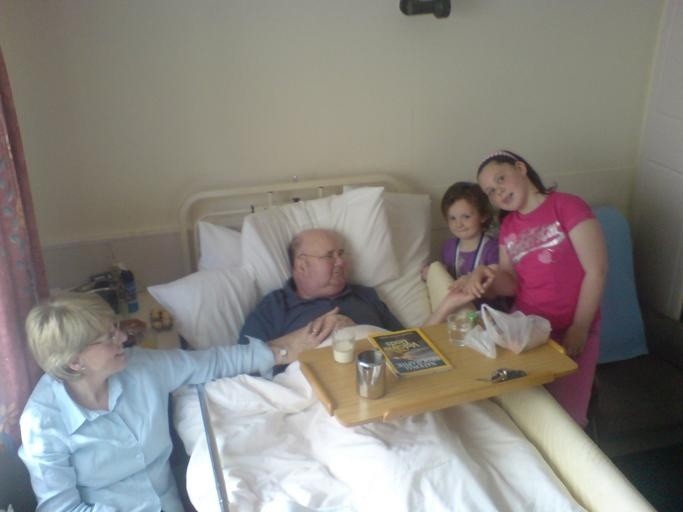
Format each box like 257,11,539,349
278,346,289,367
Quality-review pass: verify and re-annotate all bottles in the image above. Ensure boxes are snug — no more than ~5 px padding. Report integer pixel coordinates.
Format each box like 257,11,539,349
118,263,140,315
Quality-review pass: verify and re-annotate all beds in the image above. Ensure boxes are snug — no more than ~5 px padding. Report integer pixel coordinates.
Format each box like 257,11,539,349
175,173,658,512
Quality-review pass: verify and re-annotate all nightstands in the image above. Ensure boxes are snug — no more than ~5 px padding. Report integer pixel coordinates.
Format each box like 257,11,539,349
113,291,181,350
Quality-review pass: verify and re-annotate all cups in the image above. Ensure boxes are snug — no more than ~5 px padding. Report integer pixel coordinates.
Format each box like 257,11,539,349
356,348,388,401
331,329,356,364
447,313,471,349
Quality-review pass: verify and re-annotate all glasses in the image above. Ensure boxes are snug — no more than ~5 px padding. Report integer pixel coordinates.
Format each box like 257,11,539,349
90,320,120,345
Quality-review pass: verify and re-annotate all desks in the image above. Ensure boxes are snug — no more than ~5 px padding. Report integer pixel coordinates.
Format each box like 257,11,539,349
297,314,578,425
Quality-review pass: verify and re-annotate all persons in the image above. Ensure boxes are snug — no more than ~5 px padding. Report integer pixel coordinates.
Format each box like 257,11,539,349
461,149,608,433
17,288,336,512
420,182,509,314
238,227,493,383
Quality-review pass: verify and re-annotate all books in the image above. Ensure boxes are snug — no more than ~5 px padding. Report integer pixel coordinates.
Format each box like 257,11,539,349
367,328,454,377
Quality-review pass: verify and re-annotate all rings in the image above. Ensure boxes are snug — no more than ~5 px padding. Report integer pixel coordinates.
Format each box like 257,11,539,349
312,326,318,332
